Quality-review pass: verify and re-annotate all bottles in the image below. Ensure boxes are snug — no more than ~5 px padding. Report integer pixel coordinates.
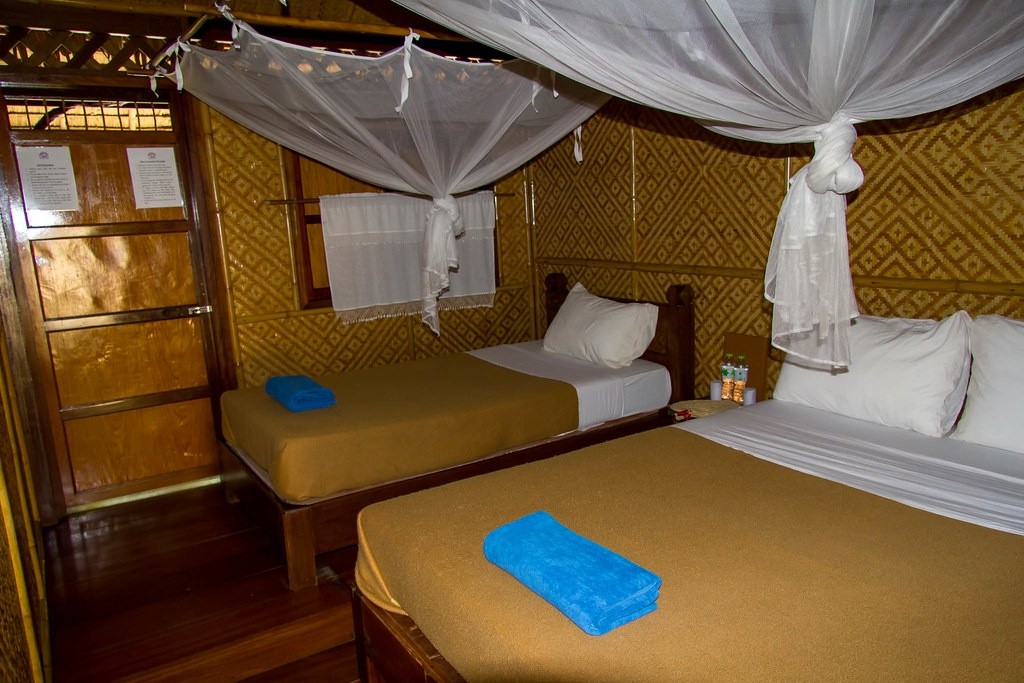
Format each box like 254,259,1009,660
721,353,735,399
733,353,748,402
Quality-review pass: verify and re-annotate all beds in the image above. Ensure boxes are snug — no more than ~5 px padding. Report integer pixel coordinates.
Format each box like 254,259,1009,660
348,401,1024,682
217,272,696,591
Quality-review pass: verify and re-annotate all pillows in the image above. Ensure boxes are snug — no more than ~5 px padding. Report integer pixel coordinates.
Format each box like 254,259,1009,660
773,309,1024,454
543,281,659,369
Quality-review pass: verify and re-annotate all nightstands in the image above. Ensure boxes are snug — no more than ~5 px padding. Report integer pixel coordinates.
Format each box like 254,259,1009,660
659,331,769,425
